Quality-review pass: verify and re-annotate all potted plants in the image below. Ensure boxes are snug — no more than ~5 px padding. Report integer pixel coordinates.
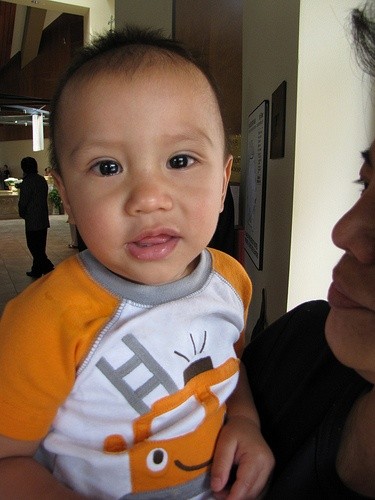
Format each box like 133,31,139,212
4,177,18,189
48,189,61,215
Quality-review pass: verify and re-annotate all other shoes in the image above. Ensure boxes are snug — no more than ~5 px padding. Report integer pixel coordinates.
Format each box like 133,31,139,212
43,267,54,274
27,271,42,278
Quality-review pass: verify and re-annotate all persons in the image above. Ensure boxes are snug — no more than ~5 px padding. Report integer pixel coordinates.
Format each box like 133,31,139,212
243,8,375,500
15,157,55,278
4,165,10,187
0,30,275,500
